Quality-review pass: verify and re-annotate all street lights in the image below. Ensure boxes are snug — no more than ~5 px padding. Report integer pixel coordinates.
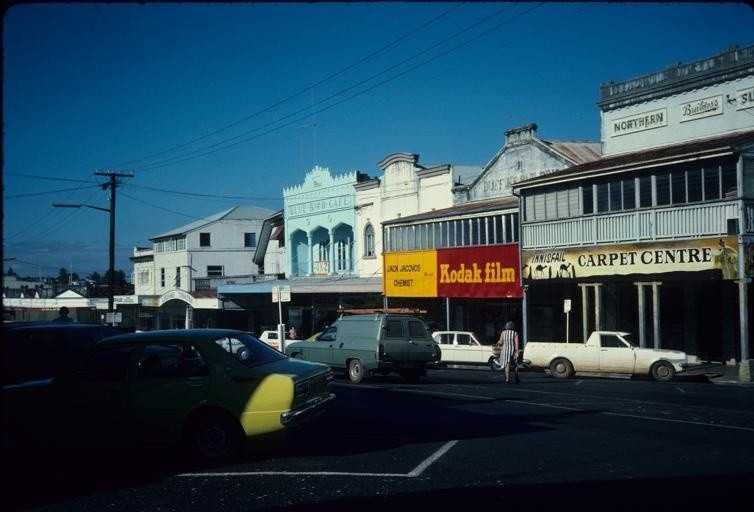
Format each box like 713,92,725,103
52,204,115,327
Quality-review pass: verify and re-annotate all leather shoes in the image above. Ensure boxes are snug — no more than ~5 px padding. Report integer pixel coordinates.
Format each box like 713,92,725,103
505,380,521,384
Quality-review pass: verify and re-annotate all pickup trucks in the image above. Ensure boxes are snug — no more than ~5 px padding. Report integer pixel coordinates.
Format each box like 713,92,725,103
523,331,688,382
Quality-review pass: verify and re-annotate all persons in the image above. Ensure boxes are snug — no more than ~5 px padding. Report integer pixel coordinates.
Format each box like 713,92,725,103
52,307,72,322
497,321,519,384
289,324,297,340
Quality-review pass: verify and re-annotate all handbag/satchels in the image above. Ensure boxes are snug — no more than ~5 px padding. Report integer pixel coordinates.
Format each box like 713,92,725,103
491,343,502,358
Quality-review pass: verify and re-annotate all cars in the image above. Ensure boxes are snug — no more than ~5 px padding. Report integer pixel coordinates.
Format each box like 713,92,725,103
431,331,504,373
